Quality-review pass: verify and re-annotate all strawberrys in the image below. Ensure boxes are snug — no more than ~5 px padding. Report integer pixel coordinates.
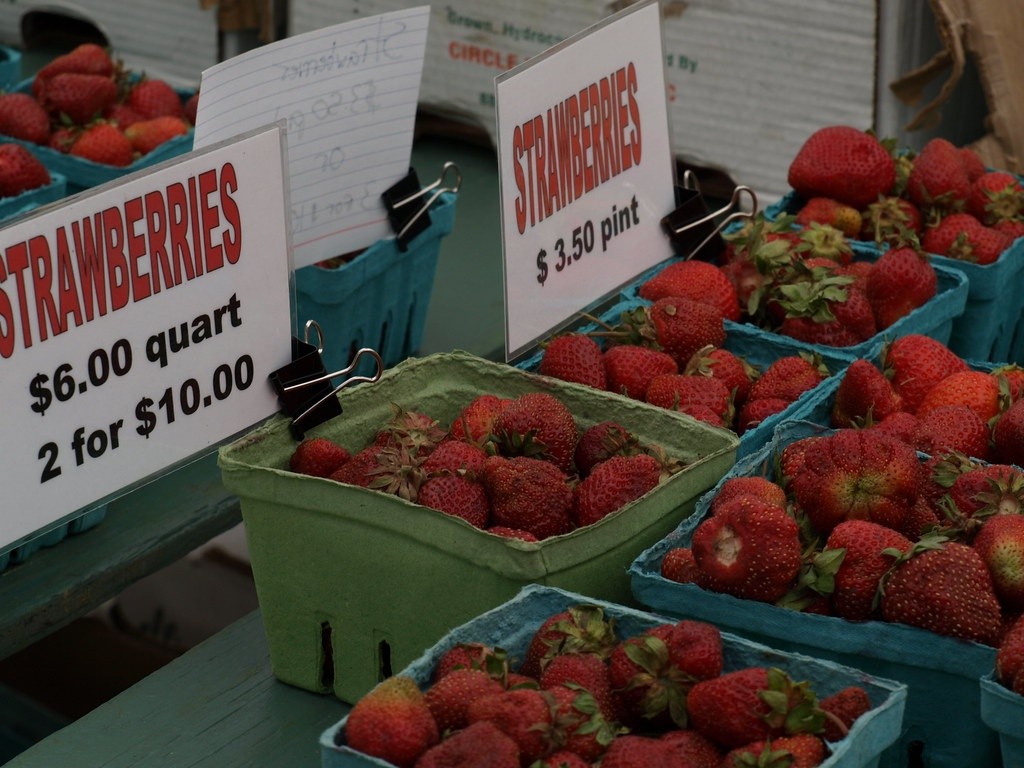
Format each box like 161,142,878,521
2,39,1024,768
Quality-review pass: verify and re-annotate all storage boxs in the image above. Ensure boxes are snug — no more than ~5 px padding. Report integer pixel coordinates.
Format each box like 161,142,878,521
0,51,204,186
0,168,65,220
290,184,457,382
219,172,1023,768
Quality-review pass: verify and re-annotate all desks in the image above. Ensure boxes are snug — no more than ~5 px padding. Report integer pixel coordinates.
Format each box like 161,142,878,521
0,436,241,654
0,604,351,768
